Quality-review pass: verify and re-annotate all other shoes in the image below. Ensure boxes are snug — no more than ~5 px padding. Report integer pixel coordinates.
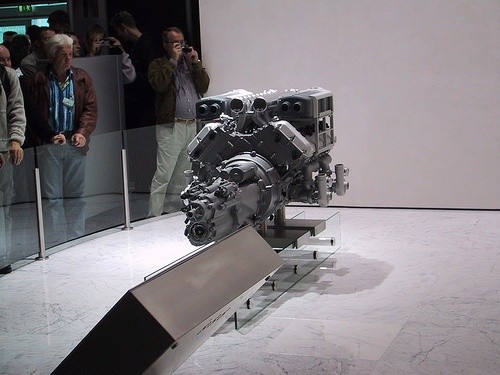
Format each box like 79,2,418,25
0,264,12,274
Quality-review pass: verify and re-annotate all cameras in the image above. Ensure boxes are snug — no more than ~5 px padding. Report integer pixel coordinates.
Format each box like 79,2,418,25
178,44,192,55
97,37,110,47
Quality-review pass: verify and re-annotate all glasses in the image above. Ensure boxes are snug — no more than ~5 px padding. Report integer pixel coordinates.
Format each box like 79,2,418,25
167,40,185,46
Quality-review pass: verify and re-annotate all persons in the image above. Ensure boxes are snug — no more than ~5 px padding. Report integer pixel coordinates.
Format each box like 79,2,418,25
147,26,210,218
28,33,99,246
0,10,159,200
0,64,27,274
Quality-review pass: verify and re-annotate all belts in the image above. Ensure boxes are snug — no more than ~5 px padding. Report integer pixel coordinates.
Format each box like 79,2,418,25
177,118,195,122
